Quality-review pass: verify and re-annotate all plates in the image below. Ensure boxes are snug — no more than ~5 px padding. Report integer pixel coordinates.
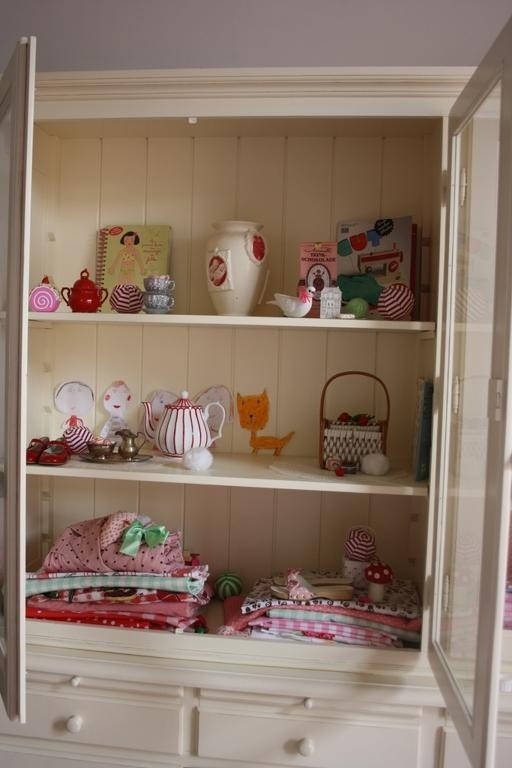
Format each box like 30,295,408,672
79,451,153,463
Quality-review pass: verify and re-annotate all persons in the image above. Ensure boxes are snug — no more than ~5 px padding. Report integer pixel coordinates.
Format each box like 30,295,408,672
106,230,147,286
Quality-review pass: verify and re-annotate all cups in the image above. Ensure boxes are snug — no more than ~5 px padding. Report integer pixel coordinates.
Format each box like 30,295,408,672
139,274,176,313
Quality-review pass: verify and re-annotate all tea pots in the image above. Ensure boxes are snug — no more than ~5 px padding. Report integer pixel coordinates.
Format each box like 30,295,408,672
115,429,147,460
62,268,108,313
141,390,226,458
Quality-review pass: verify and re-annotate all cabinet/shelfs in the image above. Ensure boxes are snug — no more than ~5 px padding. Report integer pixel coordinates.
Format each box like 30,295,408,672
1,22,508,767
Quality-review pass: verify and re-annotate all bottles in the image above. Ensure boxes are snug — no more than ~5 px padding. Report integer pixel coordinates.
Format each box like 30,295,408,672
205,221,269,315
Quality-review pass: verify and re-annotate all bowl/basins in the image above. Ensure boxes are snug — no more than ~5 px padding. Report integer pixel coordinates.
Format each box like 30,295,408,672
87,444,115,459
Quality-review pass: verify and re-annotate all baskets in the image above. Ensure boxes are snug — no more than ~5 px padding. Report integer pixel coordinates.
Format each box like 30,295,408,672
319,370,390,473
